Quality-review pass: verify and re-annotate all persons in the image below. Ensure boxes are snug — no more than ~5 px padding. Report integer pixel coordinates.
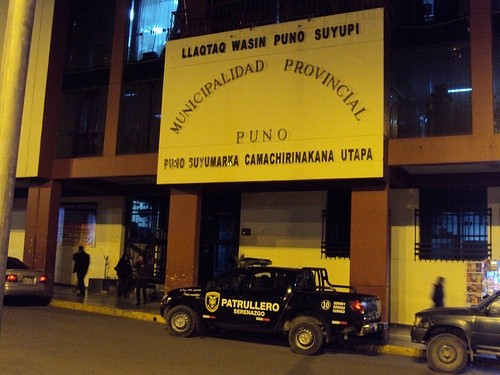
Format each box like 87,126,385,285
132,254,149,306
72,244,91,302
115,253,132,298
431,276,447,307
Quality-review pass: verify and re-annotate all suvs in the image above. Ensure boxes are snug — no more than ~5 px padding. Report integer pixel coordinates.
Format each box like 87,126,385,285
410,290,500,374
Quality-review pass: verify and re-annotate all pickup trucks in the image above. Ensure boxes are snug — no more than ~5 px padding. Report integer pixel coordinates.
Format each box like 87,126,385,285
159,257,390,355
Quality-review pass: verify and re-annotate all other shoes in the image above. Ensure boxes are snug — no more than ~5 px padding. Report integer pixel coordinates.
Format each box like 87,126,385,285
76,292,84,296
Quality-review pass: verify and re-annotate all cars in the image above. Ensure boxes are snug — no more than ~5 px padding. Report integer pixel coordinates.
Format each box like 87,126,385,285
3,256,48,306
